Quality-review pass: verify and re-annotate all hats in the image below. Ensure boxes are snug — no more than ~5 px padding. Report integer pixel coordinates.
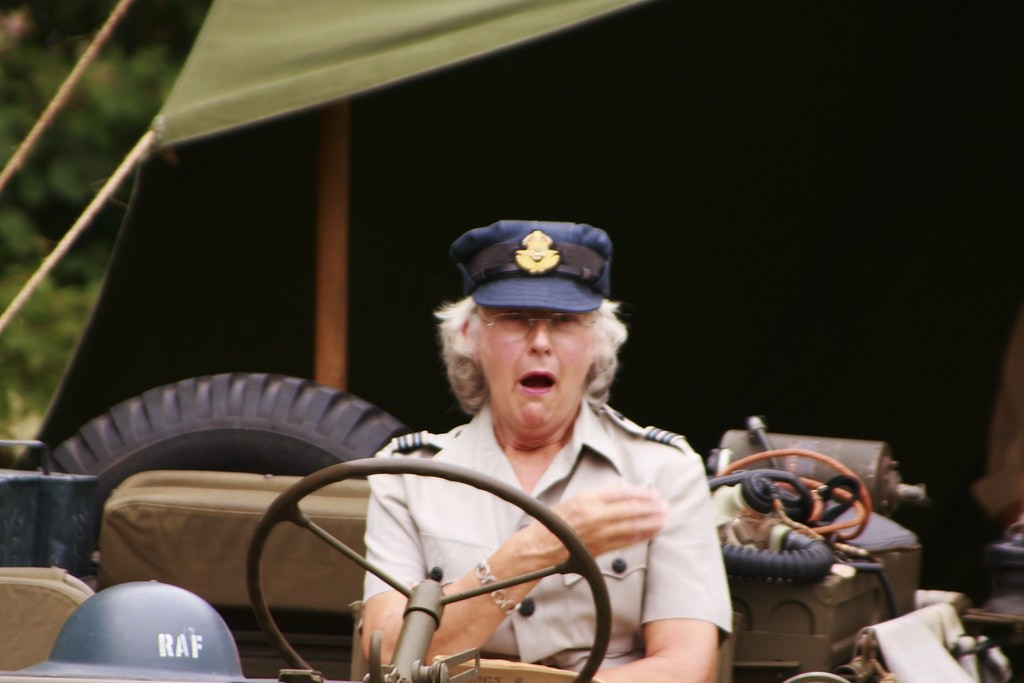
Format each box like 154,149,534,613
447,219,612,313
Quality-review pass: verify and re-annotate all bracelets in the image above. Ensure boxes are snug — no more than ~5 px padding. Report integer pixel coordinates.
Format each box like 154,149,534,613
474,559,521,615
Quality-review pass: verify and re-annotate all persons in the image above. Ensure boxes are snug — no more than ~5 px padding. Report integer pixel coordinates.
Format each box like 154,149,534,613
359,220,734,683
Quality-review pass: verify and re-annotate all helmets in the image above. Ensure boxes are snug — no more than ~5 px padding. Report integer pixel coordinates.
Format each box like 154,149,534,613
14,580,249,683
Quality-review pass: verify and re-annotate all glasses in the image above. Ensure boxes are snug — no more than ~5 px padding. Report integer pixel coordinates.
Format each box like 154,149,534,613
478,309,596,340
